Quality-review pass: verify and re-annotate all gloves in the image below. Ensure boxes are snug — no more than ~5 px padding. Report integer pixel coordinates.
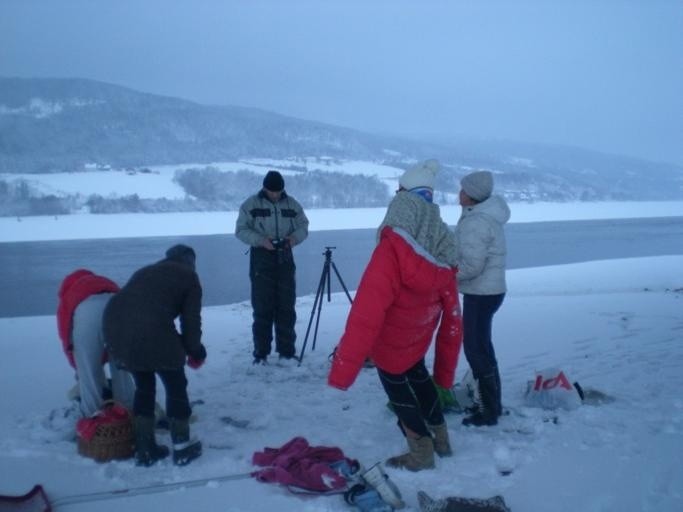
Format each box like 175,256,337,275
187,354,204,368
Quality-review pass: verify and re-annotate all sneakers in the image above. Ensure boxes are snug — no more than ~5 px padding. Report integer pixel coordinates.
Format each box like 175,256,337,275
277,355,301,369
248,358,265,375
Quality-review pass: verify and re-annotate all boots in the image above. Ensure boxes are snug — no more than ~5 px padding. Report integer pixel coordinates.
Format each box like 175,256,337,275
136,416,169,466
461,372,498,427
425,418,453,459
385,421,435,472
170,411,202,466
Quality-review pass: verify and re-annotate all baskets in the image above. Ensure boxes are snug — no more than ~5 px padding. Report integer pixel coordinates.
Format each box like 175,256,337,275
77,400,135,464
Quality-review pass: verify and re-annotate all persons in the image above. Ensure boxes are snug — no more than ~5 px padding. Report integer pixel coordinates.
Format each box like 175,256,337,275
452,170,511,426
56,270,136,415
325,159,462,472
102,244,206,468
234,169,309,365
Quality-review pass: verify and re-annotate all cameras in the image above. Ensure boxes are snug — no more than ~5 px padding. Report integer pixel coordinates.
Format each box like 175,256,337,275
272,237,289,250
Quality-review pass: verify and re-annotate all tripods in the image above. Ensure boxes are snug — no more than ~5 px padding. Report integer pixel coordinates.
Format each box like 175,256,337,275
298,246,353,368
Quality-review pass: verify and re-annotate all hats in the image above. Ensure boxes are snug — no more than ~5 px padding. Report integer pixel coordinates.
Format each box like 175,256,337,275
263,170,284,192
398,158,440,193
166,244,196,270
461,171,494,203
